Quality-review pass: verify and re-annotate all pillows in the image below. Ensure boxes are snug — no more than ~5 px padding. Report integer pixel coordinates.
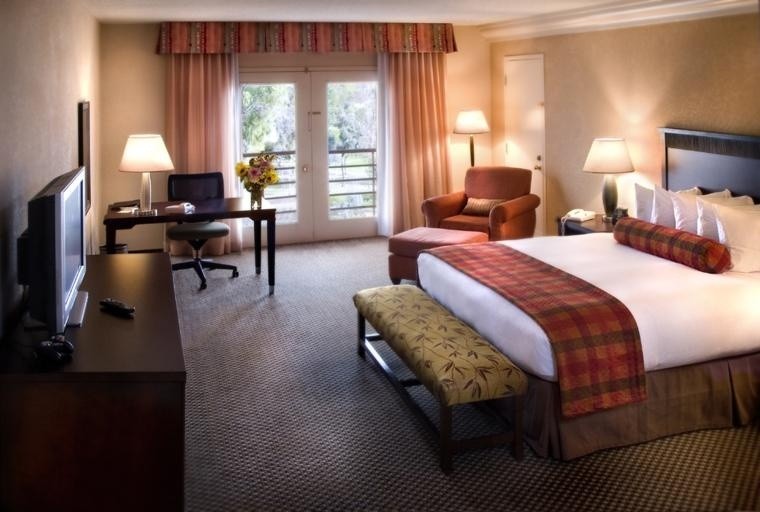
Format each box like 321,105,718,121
461,196,507,216
613,182,760,275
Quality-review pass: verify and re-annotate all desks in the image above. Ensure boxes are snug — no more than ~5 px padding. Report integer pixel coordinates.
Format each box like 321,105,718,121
105,195,277,296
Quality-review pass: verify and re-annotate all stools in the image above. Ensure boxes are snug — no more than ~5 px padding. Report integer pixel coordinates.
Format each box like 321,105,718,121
387,226,488,285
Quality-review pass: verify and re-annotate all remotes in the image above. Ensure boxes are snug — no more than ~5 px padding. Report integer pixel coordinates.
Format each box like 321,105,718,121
99,298,135,315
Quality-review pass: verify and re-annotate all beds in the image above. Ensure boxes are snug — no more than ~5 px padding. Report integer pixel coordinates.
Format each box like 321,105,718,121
414,126,760,462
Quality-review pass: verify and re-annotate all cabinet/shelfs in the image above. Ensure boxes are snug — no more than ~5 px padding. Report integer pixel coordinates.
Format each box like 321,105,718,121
1,252,187,511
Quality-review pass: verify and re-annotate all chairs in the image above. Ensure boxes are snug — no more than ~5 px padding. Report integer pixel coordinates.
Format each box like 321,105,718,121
166,171,239,291
421,165,541,242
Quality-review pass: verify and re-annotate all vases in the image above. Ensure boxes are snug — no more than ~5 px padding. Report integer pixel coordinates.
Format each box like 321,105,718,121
251,191,262,210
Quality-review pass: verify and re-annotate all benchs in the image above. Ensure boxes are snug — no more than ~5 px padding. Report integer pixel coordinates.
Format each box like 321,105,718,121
353,284,529,475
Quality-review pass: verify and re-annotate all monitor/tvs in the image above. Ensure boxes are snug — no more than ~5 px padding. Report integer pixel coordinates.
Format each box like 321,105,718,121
17,165,89,335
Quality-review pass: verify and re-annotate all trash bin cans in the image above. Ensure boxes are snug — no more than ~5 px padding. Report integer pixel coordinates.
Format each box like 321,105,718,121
99,244,128,255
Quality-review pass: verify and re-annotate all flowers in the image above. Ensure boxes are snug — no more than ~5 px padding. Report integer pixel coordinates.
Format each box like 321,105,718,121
235,150,282,207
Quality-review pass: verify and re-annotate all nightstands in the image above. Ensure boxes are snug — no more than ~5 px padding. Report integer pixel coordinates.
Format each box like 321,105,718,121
553,214,613,236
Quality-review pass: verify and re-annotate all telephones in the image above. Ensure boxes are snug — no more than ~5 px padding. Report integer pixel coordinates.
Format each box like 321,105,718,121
563,209,595,222
165,202,195,214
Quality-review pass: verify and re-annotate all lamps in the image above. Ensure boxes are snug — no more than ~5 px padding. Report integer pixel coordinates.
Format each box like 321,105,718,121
118,134,175,213
582,137,635,222
451,109,490,167
